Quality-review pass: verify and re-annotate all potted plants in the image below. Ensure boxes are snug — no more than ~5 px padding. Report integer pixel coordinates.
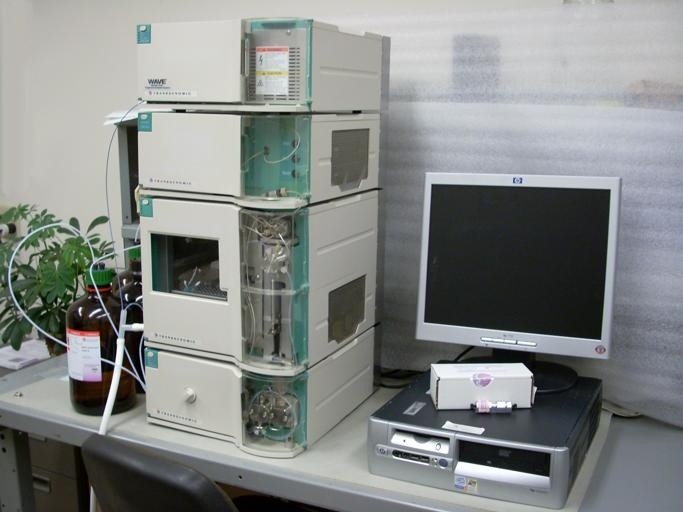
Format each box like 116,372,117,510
0,204,116,358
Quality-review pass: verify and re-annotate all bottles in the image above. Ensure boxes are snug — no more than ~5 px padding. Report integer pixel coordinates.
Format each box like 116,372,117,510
65,270,142,417
109,244,146,398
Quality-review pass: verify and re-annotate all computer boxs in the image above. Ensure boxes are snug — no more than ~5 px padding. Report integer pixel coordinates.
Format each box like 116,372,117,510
367,357,603,510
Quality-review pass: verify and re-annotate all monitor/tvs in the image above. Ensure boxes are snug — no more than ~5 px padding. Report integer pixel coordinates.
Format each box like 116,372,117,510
414,172,622,393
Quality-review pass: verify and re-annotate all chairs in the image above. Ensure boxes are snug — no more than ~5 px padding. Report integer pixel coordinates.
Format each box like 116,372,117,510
81,435,333,511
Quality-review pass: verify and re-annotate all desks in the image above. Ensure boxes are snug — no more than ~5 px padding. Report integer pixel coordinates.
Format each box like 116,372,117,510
0,350,675,510
